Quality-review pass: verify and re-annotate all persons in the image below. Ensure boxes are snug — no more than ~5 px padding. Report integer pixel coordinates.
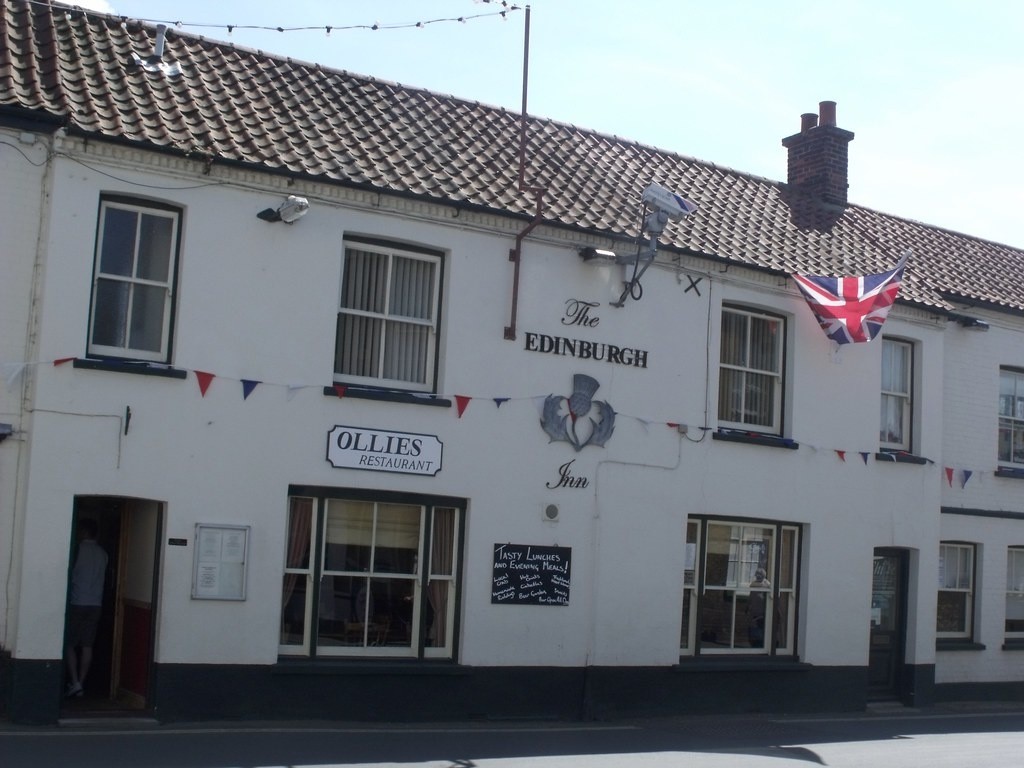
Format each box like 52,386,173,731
742,568,781,647
61,520,111,699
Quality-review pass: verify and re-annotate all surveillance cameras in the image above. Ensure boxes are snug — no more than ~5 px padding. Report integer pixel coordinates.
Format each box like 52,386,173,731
639,181,697,225
279,197,309,223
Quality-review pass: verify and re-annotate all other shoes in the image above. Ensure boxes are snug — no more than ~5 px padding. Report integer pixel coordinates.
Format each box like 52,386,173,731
64,682,82,696
71,689,85,706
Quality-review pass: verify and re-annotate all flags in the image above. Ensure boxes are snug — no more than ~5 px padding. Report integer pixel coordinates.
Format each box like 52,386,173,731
793,259,905,345
